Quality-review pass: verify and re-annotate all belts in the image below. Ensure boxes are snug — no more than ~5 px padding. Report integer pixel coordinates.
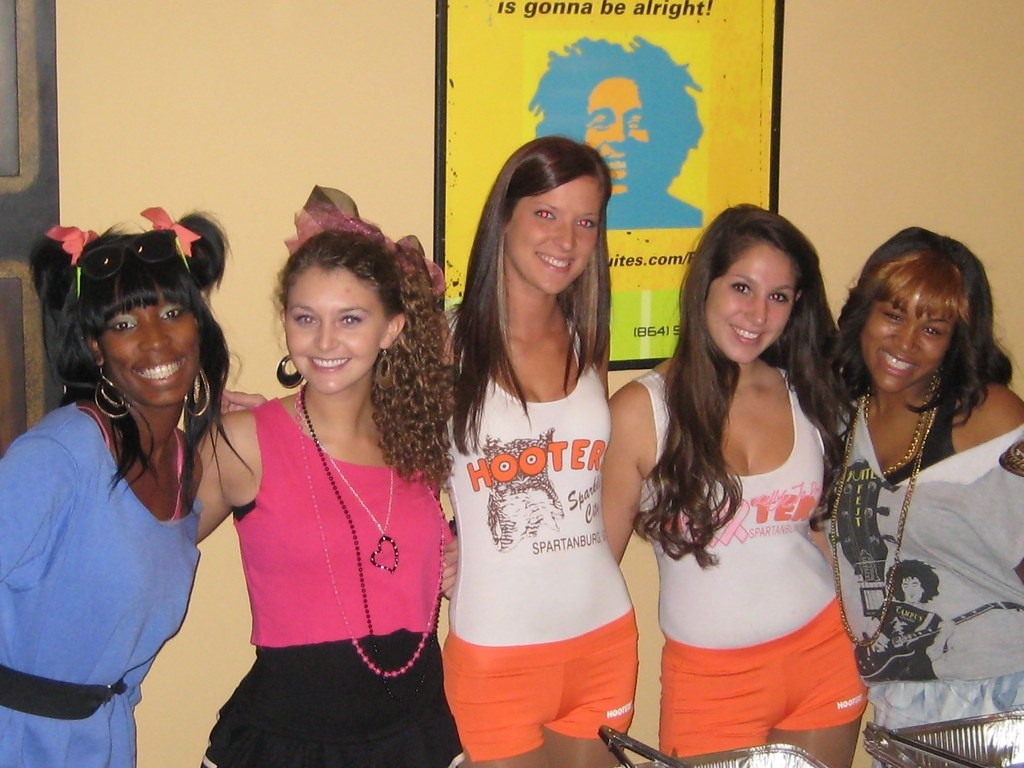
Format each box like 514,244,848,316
0,664,128,720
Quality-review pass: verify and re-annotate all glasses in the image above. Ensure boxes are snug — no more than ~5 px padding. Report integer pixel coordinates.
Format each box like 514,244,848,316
76,230,191,301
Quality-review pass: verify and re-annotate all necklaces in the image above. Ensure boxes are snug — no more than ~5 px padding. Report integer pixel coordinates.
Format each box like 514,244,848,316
294,384,446,702
831,372,944,647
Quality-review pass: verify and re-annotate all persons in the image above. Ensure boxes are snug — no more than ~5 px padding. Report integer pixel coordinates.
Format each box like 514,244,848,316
0,208,229,768
219,136,658,768
194,229,463,768
442,201,868,768
831,225,1024,768
